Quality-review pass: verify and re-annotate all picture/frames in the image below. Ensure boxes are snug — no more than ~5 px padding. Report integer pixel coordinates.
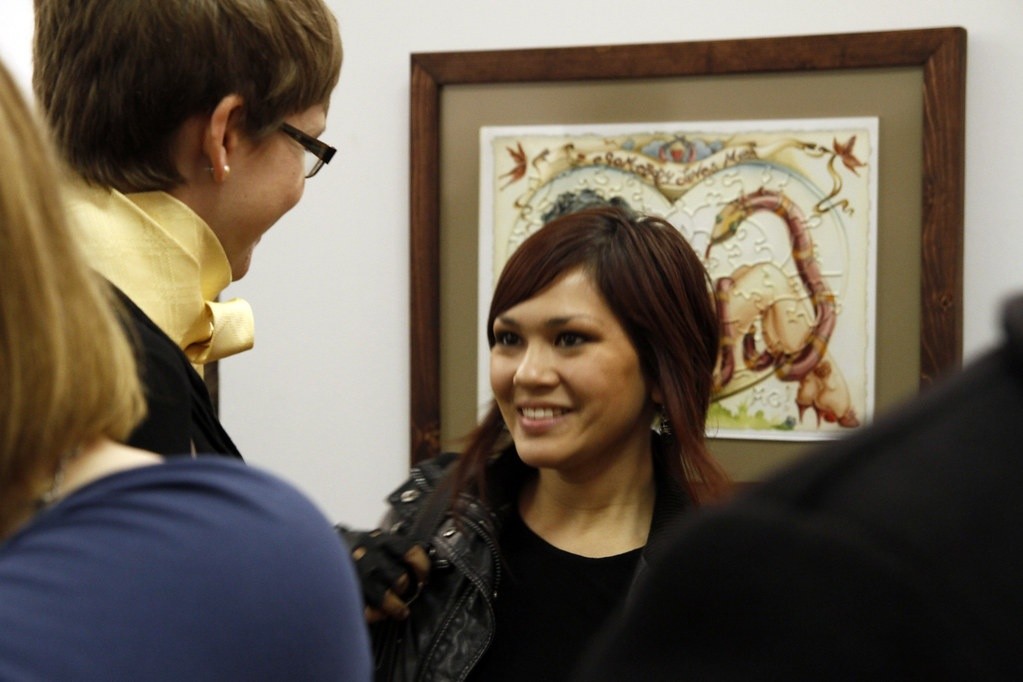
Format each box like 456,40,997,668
405,24,973,485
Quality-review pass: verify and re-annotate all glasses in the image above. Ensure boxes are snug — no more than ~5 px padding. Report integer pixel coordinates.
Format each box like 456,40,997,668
281,120,337,179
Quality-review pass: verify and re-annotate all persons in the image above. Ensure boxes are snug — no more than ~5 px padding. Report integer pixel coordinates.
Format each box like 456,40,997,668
34,0,348,458
326,206,731,682
0,58,373,682
